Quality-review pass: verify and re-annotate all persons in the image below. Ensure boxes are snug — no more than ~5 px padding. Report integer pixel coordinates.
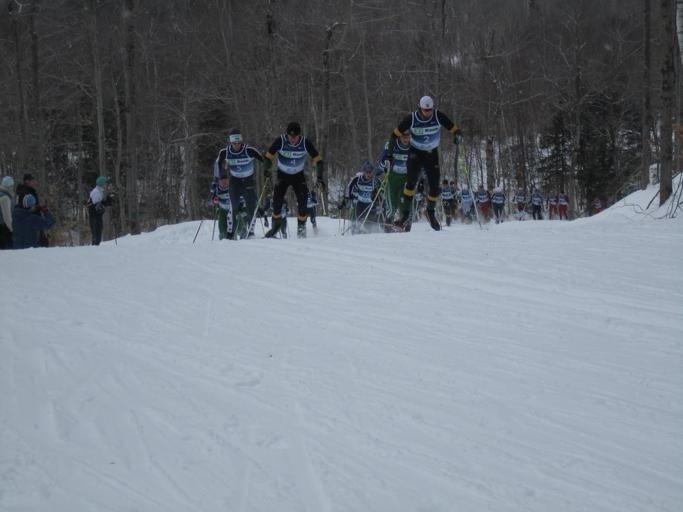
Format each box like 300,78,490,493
87,176,117,246
1,173,56,248
590,194,603,214
344,95,463,236
545,190,572,221
207,121,326,240
457,185,546,224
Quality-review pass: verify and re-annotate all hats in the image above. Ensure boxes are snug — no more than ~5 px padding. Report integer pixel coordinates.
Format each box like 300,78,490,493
362,161,373,170
230,129,243,143
96,176,110,184
2,174,36,208
287,123,300,136
420,96,433,109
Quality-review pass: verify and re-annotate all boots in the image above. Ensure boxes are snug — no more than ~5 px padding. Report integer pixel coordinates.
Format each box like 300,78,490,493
425,209,440,231
394,211,413,231
297,219,307,236
265,217,282,237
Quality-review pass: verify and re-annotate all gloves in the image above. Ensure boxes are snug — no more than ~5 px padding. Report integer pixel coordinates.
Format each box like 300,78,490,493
455,132,464,145
316,178,326,192
